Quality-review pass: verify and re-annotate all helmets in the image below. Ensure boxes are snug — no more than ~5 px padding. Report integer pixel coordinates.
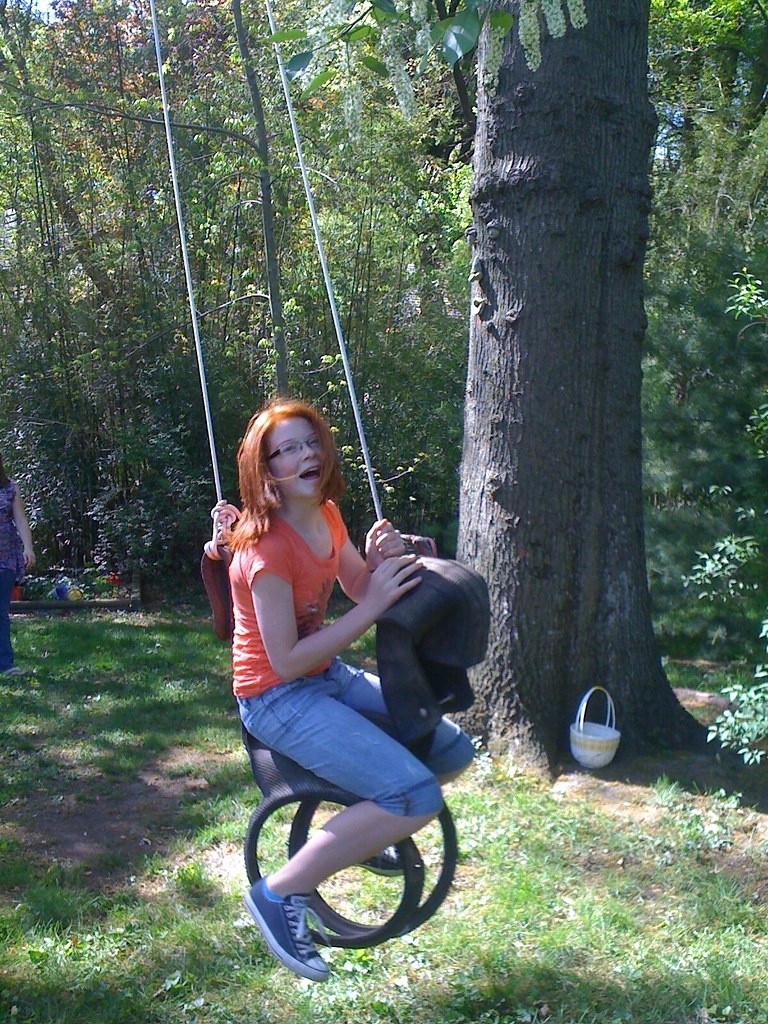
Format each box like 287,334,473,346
66,588,83,601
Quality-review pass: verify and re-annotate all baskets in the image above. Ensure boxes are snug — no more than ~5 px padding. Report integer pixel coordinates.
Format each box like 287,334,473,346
570,687,621,768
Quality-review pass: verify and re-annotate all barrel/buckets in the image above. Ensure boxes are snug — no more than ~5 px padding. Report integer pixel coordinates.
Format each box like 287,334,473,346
47,587,66,601
10,587,24,601
66,588,83,600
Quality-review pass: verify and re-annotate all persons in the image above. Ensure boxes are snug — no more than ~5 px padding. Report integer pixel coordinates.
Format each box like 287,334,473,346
226,402,477,982
0,453,37,675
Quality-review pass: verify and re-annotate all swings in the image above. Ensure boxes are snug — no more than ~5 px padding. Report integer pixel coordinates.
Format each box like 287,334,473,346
140,1,460,949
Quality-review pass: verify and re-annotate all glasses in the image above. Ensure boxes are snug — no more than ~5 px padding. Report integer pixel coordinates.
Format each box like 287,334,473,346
267,437,318,461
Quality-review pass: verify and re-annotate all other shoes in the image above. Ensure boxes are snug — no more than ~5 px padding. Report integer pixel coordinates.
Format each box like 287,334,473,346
6,666,25,676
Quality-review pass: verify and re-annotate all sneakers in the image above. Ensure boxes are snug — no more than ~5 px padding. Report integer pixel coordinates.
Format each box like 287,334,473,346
352,847,405,877
244,874,330,982
